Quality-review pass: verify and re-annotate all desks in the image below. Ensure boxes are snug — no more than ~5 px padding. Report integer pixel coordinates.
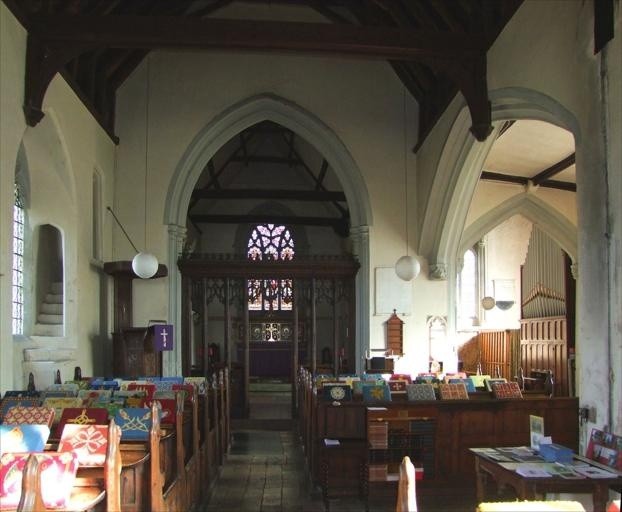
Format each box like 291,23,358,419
469,444,621,511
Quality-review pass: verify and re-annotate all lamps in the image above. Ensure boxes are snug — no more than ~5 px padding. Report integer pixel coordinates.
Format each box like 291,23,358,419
394,55,420,280
132,48,159,279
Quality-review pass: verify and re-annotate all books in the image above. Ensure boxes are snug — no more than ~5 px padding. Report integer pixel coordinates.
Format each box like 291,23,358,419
469,415,621,483
313,371,524,402
0,375,207,512
358,406,438,498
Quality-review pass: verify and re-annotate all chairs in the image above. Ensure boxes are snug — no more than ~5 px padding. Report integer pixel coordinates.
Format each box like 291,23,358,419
316,405,371,511
1,377,208,512
313,373,525,400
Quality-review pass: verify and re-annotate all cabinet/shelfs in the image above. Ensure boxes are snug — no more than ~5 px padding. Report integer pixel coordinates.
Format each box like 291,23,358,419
386,309,403,355
